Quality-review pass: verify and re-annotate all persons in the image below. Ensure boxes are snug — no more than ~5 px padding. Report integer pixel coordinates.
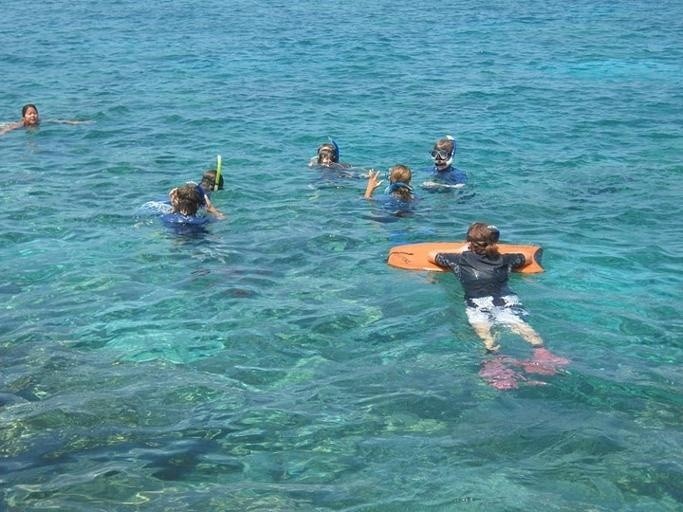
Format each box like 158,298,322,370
308,144,351,168
427,223,543,356
365,165,424,211
0,104,97,136
415,134,467,193
198,170,224,191
157,182,225,240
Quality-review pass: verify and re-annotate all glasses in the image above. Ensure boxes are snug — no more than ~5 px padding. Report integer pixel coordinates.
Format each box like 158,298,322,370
428,150,449,161
